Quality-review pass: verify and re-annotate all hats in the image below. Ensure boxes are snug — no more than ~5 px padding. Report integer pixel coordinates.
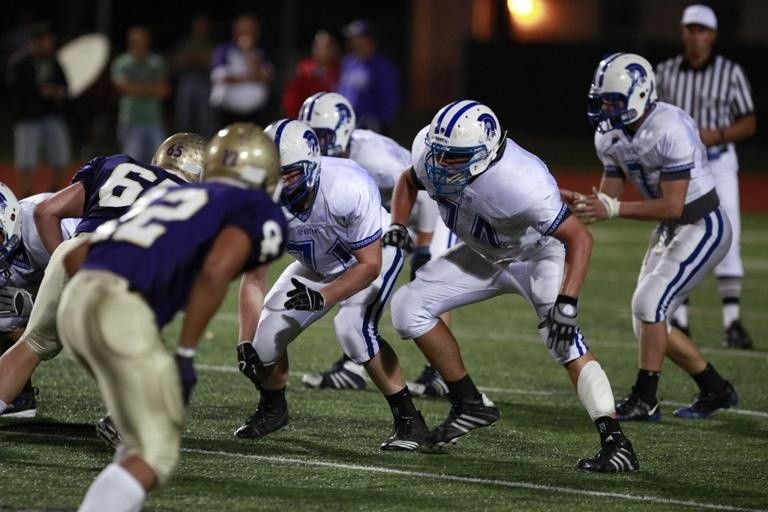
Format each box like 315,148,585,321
344,22,372,36
131,27,154,49
681,5,717,32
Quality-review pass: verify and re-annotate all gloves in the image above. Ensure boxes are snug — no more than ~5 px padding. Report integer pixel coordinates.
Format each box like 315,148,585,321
0,287,36,318
380,223,414,254
175,348,197,407
236,341,266,391
538,294,579,353
410,246,431,281
284,278,324,312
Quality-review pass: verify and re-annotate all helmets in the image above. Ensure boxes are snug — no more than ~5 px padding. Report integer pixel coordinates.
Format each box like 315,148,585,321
425,100,501,197
298,92,355,157
0,182,21,265
151,131,207,184
199,122,281,204
588,53,658,135
262,119,321,213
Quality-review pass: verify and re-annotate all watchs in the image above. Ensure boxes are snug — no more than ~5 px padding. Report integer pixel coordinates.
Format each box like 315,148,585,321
718,126,726,138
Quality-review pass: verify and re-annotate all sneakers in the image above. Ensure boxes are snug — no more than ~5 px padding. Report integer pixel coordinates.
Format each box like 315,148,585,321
380,411,430,451
301,359,368,390
424,390,500,449
0,387,39,418
234,398,290,438
673,380,738,419
96,416,122,449
722,321,752,350
576,432,640,473
405,363,449,398
615,395,661,422
670,318,690,338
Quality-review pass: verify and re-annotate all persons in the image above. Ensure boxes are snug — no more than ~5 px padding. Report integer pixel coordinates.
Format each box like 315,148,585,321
656,5,755,350
559,53,738,423
56,122,288,512
298,92,463,399
209,17,274,127
109,25,170,163
172,16,213,138
281,30,341,119
0,180,82,419
382,100,640,473
234,119,430,451
1,34,68,199
338,21,399,137
0,132,206,449
